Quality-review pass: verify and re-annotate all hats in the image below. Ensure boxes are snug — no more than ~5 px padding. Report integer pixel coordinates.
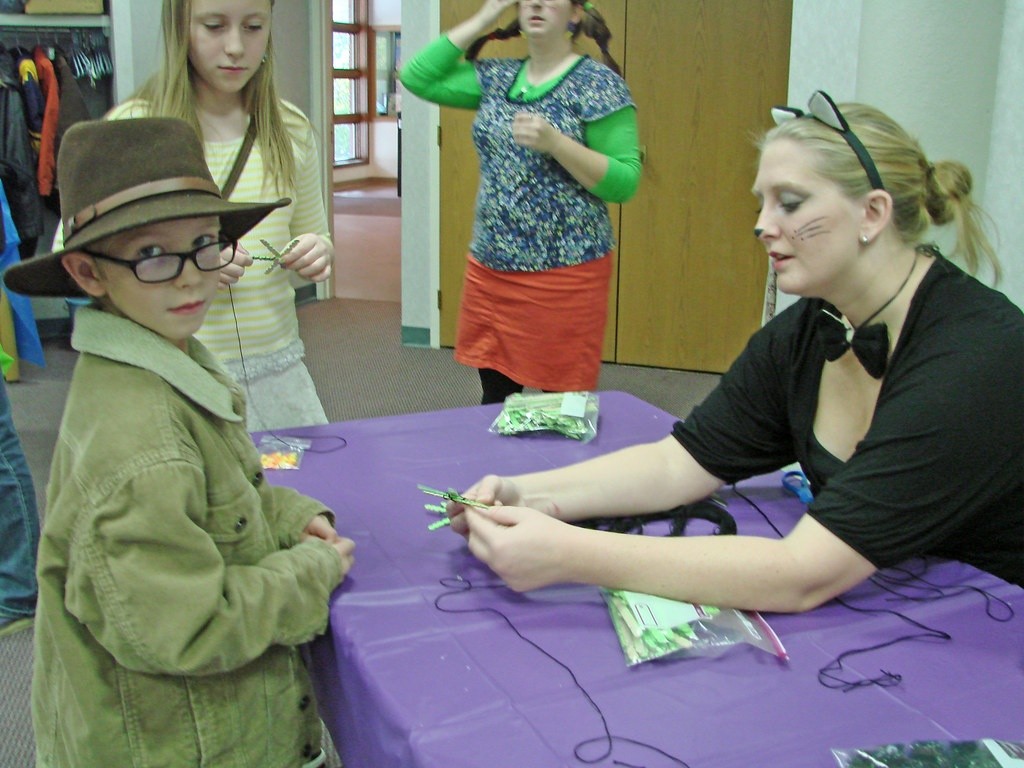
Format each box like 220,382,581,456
3,116,293,298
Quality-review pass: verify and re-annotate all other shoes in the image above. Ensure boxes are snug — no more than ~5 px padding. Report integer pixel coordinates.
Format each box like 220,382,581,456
0,615,35,636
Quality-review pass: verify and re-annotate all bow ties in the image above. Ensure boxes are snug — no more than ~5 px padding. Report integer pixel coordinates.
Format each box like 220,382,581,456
813,307,891,381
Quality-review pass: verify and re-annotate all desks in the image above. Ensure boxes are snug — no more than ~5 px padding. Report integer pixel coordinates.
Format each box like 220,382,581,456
244,391,1024,768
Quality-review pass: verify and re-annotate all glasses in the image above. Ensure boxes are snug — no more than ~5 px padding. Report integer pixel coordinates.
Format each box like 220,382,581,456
80,230,238,283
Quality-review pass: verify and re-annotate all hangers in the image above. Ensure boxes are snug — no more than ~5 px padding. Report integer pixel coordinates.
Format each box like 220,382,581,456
14,29,113,87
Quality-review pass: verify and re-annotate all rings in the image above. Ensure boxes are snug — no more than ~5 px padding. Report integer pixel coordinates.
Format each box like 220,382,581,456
529,113,532,119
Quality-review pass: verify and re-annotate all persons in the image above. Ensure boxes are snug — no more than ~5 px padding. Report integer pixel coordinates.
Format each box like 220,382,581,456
0,179,41,642
55,0,337,431
446,104,1024,613
0,116,357,768
398,0,644,404
